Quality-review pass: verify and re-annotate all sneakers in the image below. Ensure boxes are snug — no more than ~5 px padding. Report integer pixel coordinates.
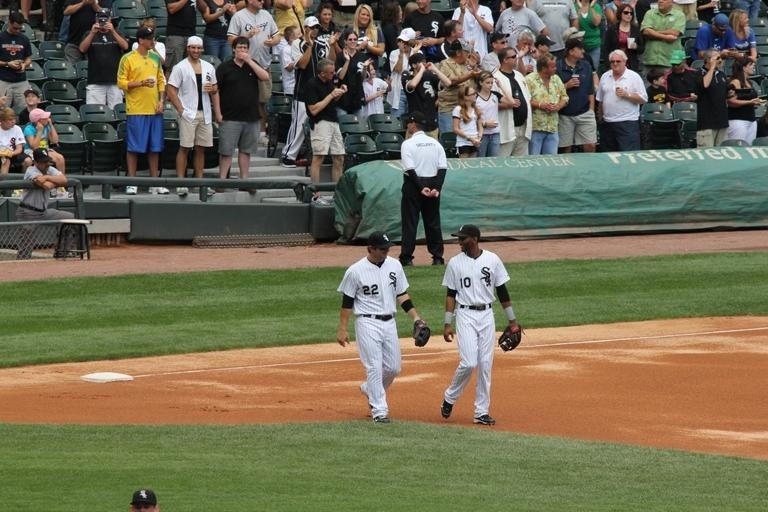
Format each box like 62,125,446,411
175,186,189,197
374,413,390,423
148,185,170,195
280,155,298,168
238,187,257,194
440,398,455,419
53,251,82,260
192,185,225,196
472,413,496,425
125,185,138,195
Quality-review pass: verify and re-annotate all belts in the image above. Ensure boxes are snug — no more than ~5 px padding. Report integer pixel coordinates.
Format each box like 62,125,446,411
359,312,397,322
19,202,46,213
459,303,492,311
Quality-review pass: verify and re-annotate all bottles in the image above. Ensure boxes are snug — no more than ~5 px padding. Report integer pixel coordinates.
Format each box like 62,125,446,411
514,89,520,99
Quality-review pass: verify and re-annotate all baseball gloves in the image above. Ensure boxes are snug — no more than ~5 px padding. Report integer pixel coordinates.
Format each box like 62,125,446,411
413,320,431,347
498,322,525,352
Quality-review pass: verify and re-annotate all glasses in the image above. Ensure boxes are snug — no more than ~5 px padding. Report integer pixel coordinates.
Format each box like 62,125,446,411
609,60,622,63
348,37,357,42
621,10,635,15
506,54,517,60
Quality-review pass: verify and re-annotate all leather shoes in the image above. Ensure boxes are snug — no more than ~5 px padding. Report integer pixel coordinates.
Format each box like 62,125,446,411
431,258,445,265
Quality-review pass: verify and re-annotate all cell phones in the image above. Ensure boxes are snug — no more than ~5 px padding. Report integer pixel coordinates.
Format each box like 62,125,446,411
529,48,536,53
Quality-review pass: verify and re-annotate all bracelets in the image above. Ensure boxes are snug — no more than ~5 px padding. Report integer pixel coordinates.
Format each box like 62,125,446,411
502,306,518,322
442,312,455,324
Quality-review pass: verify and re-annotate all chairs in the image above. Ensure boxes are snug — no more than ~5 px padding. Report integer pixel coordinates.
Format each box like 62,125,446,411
0,0,768,198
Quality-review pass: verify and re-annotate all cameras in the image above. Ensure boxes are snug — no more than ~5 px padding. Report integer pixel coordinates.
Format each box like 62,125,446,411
99,10,108,26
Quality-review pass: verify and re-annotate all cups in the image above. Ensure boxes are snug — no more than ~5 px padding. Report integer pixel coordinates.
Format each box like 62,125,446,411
571,74,580,87
148,76,155,88
626,37,636,48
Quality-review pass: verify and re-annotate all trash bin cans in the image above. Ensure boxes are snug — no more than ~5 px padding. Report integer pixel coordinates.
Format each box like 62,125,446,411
311,204,336,242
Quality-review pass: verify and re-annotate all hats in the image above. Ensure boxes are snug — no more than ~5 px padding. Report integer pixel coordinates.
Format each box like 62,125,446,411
185,34,204,49
409,52,428,64
28,108,52,123
450,37,469,51
561,26,586,49
136,26,154,39
536,34,556,46
366,230,394,249
396,26,416,43
489,30,511,43
450,223,481,243
129,488,158,507
33,147,52,162
715,13,730,28
670,49,686,66
304,15,321,30
96,8,110,18
403,111,429,125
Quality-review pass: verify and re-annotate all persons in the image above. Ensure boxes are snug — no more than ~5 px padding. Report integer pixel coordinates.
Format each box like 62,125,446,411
1,0,166,173
16,147,75,260
166,0,393,185
380,0,648,158
439,225,521,425
117,27,170,195
168,36,222,196
640,1,766,150
397,109,448,266
336,231,427,424
128,489,159,512
211,36,269,194
